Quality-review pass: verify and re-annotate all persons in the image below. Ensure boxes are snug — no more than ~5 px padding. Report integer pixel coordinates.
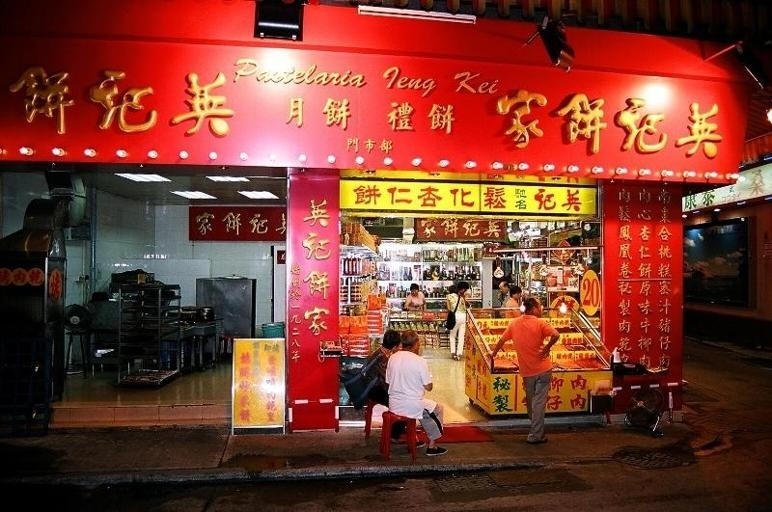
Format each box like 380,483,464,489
366,329,405,444
446,282,469,360
505,286,523,307
385,330,448,456
496,281,511,308
404,283,426,311
488,297,560,443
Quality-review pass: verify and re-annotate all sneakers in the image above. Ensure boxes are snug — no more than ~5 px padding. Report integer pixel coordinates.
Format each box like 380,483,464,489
417,441,447,456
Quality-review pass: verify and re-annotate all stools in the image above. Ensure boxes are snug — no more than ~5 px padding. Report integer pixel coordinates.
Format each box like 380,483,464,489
218,333,240,365
379,411,417,462
364,400,388,440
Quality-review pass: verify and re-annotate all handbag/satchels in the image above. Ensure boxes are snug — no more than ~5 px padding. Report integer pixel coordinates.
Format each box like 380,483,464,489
447,311,455,329
344,354,385,410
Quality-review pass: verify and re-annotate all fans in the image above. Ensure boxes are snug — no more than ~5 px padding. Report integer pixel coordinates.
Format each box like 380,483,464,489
63,304,92,333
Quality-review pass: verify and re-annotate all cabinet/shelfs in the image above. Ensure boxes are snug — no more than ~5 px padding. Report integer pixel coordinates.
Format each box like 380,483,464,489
341,244,390,359
372,240,483,349
464,309,614,416
117,281,181,389
513,221,603,308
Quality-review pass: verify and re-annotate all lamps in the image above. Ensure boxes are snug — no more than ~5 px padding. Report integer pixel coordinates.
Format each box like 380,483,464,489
524,11,575,73
494,255,505,280
